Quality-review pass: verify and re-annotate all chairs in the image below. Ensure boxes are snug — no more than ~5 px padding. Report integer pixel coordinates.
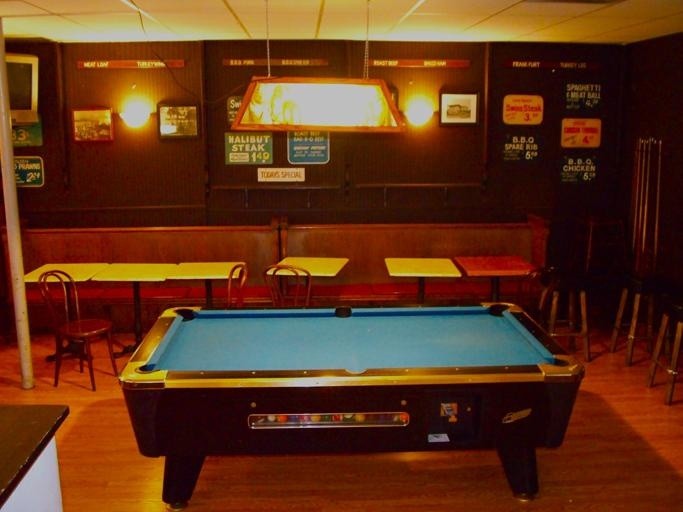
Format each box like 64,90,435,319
221,262,251,310
260,262,315,309
38,268,126,396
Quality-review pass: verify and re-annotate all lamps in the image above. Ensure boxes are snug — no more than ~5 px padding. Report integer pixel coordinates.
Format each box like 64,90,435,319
228,1,406,135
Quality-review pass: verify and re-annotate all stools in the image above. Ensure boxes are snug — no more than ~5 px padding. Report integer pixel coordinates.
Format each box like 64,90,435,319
647,297,683,406
606,265,677,365
534,263,596,367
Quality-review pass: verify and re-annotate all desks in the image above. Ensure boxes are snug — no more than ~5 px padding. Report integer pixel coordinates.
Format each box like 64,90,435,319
454,250,540,306
384,253,463,309
93,261,179,359
21,262,96,366
162,260,247,310
112,302,586,512
0,401,70,512
265,255,351,310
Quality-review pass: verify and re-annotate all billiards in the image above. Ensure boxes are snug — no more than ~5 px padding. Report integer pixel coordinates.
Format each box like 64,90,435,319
392,414,400,424
258,414,366,424
400,414,408,424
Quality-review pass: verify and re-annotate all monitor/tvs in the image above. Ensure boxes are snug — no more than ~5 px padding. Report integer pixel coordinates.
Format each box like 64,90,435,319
5,53,40,125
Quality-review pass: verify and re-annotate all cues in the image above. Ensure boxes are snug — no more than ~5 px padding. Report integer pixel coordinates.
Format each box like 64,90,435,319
629,138,662,264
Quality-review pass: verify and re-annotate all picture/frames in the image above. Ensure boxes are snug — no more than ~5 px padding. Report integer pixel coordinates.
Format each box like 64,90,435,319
158,100,201,141
438,88,481,128
70,106,116,144
